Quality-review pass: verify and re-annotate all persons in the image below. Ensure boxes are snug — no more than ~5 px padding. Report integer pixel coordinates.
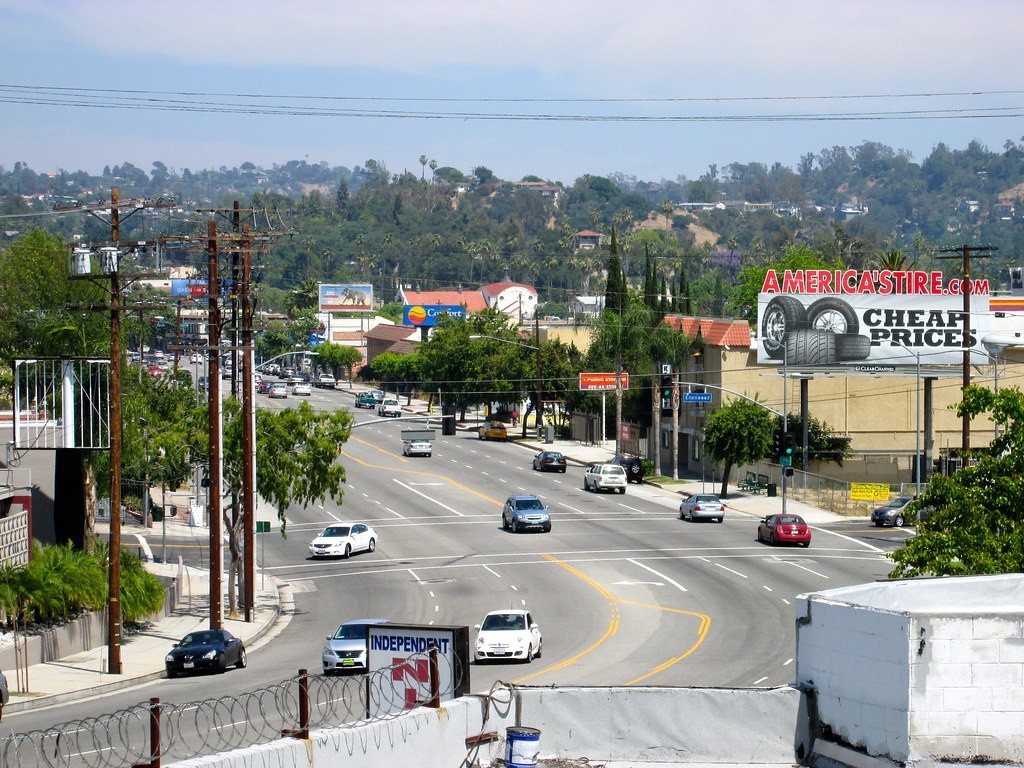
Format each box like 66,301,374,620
511,408,518,425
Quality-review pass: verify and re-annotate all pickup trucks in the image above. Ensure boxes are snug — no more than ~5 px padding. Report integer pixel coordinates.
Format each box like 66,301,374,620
355,392,377,409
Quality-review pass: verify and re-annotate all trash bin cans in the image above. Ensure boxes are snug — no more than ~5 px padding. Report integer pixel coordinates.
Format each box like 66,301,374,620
768,483,776,495
257,521,271,531
153,507,163,522
546,426,554,444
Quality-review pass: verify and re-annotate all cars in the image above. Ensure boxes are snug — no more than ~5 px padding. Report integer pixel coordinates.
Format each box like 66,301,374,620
502,494,551,532
126,344,336,399
584,464,627,494
478,421,507,442
322,618,393,676
378,398,402,417
308,523,379,560
403,439,433,457
367,387,385,404
758,514,811,547
533,451,566,473
680,493,725,523
473,609,542,664
165,629,247,678
0,669,9,722
871,496,932,527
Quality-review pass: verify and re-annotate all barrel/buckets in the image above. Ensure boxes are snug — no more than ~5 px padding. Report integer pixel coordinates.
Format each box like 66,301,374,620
507,726,541,768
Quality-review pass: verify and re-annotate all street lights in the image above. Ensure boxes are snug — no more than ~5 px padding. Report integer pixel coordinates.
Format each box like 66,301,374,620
251,340,321,624
468,334,543,441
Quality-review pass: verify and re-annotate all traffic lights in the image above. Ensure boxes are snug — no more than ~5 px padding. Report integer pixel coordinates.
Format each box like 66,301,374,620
773,429,794,456
660,374,672,398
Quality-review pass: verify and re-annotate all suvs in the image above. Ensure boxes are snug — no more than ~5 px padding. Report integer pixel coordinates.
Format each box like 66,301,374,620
612,454,645,484
